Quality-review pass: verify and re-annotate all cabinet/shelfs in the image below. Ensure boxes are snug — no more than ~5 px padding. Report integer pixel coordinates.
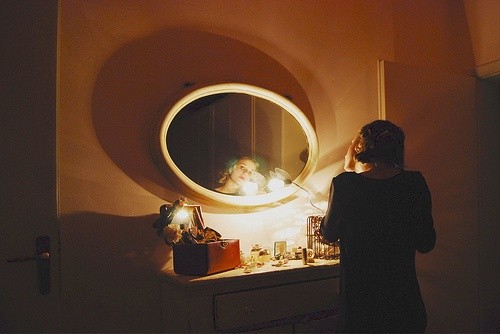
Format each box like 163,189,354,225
166,94,281,189
160,259,340,334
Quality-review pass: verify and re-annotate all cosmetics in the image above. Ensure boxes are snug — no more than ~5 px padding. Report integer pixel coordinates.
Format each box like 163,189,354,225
244,241,315,273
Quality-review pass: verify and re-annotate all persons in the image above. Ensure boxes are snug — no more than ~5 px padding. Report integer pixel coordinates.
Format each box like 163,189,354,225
319,120,436,334
213,156,259,197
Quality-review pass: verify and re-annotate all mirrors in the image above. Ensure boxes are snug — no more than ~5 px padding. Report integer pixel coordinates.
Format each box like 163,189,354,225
160,83,319,205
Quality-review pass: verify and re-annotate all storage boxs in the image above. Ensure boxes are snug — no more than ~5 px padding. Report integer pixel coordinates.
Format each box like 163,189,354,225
160,203,240,275
